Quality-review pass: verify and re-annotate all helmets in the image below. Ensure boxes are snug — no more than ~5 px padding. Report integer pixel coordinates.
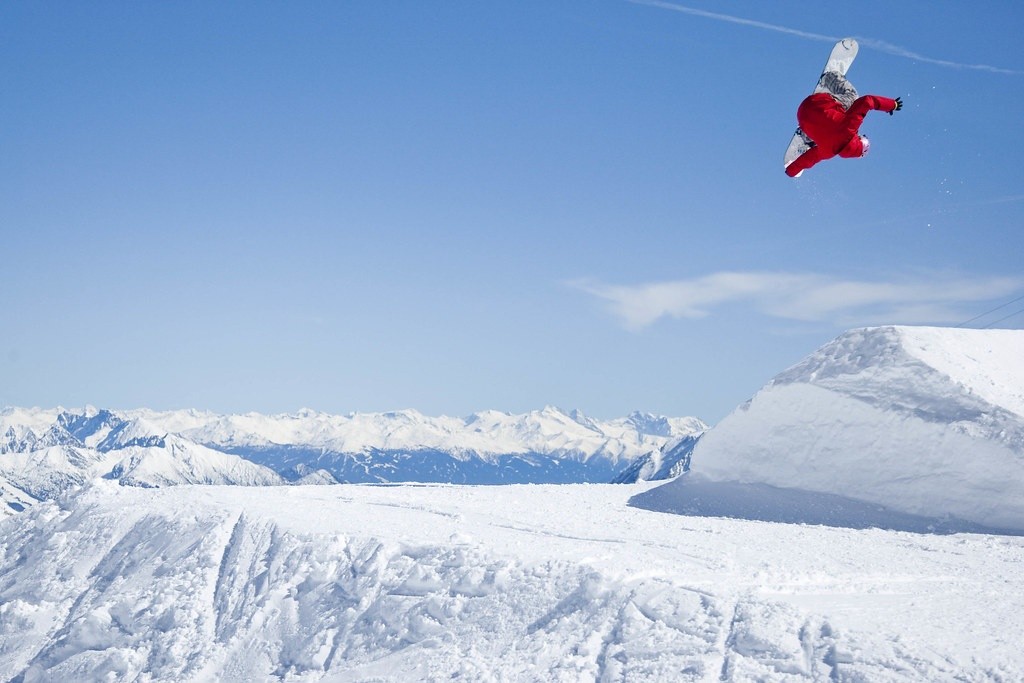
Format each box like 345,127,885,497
858,134,871,158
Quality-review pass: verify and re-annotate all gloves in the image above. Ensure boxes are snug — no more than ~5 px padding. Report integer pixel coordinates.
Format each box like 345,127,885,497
890,96,903,116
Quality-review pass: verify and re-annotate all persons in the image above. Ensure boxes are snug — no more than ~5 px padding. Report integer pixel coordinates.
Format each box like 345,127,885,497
783,67,905,178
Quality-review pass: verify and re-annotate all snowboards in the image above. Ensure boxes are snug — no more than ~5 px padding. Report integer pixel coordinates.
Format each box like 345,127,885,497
784,38,860,177
